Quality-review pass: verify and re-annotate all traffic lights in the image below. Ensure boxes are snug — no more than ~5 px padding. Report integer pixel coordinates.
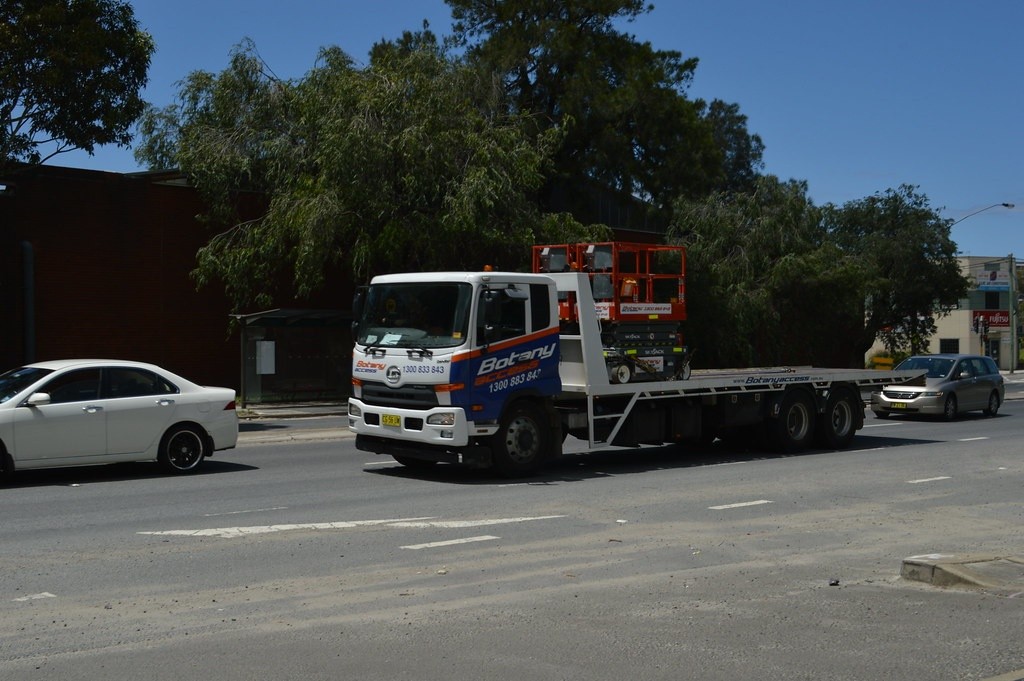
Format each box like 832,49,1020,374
973,317,980,336
983,319,989,335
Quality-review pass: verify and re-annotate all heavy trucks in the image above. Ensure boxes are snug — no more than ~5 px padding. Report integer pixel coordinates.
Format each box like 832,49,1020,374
343,264,931,484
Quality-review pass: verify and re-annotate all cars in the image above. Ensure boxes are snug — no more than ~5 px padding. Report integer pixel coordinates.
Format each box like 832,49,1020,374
872,353,1005,424
0,357,240,486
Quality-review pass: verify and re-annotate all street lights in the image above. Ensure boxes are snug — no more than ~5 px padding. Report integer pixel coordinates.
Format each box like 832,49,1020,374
911,202,1017,353
